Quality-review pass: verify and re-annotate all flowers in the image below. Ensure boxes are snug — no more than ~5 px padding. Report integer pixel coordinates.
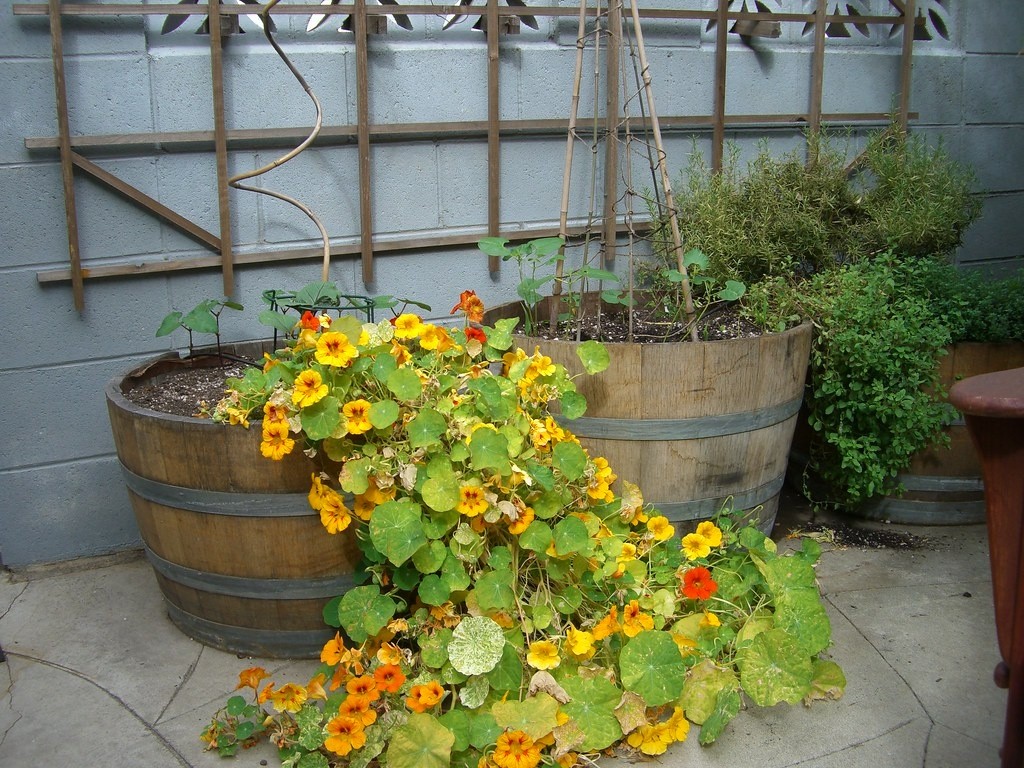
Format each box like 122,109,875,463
197,289,845,768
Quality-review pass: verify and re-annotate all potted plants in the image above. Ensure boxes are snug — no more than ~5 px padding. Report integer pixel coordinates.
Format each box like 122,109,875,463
470,234,815,538
814,253,1024,526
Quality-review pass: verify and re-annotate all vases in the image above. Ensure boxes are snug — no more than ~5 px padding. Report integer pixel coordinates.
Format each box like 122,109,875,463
104,340,368,661
